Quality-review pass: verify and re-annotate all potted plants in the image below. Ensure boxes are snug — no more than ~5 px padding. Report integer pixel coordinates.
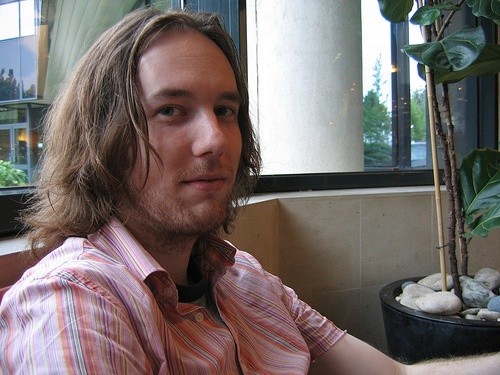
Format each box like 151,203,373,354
379,0,499,366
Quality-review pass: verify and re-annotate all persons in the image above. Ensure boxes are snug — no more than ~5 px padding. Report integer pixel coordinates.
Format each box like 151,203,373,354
0,6,500,375
